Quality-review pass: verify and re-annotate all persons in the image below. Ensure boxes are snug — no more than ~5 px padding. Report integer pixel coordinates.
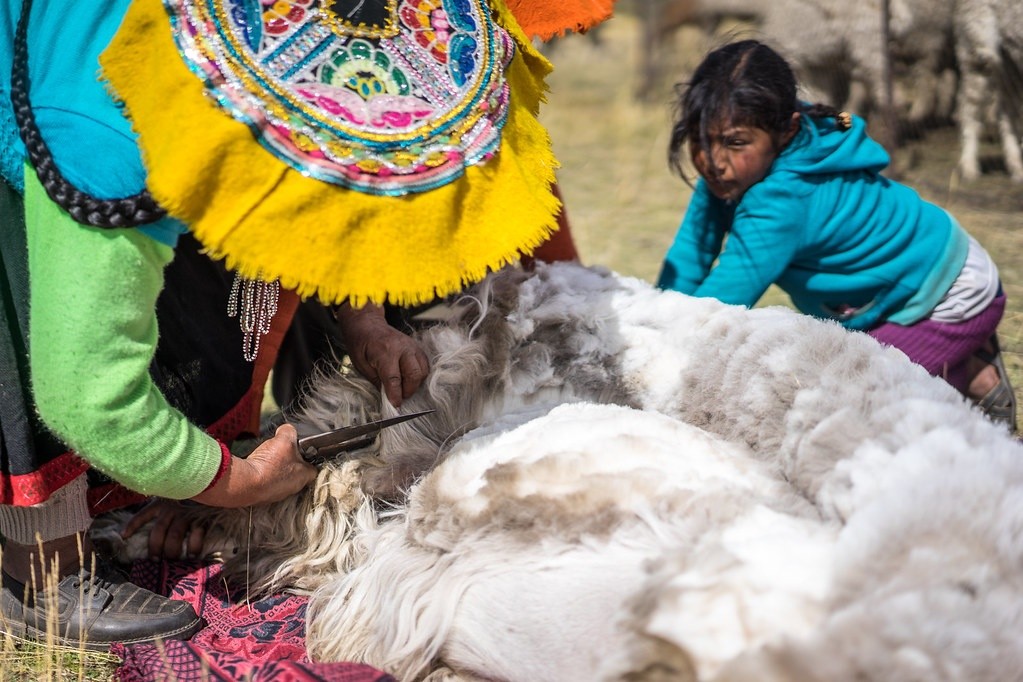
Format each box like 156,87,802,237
655,39,1018,433
0,0,615,651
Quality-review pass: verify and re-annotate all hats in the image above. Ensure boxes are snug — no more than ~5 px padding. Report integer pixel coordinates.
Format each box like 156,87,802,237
99,0,564,309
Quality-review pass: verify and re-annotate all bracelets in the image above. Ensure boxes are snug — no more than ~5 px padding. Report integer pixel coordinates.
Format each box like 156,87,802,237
330,298,351,320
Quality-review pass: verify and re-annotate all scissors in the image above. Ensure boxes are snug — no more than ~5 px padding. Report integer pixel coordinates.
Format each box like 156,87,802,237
296,406,439,465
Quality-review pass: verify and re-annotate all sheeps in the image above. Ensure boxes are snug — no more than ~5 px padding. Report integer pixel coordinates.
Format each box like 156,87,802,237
581,0,1023,173
79,253,1023,682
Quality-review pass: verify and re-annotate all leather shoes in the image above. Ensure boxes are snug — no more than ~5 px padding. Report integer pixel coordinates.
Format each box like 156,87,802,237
0,557,204,651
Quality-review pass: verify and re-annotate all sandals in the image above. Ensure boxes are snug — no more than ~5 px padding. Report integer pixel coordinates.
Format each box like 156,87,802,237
950,331,1015,437
